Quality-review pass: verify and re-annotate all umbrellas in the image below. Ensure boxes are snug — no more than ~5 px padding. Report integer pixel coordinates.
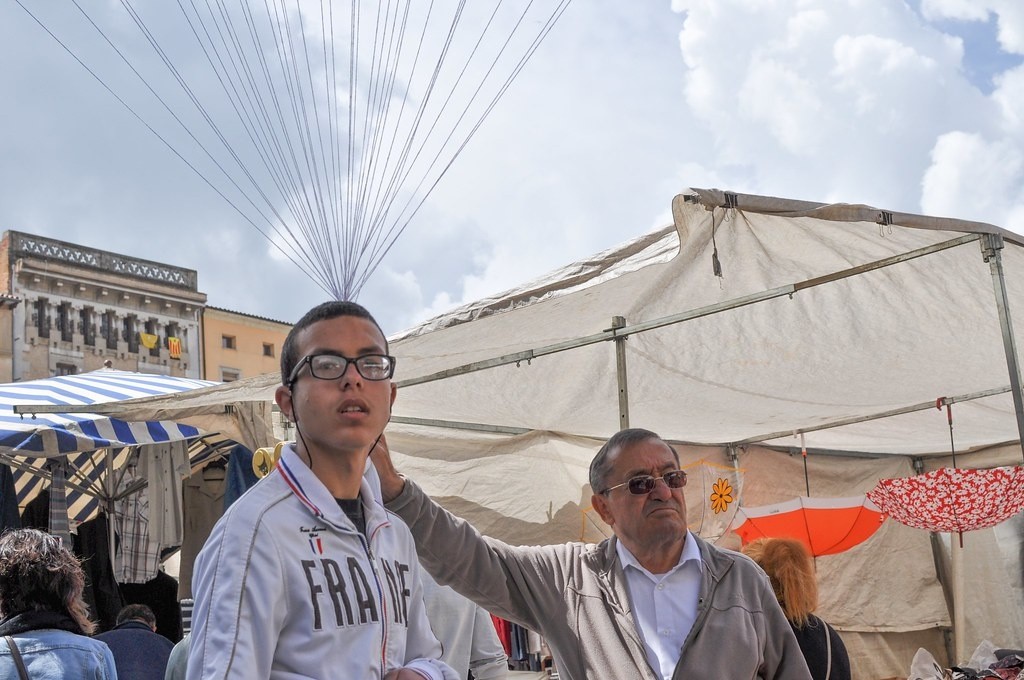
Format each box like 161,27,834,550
0,362,239,582
863,396,1023,548
727,427,890,573
575,459,746,549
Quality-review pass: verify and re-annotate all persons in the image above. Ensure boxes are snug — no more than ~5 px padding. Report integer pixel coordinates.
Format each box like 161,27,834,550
738,538,851,680
0,529,118,680
185,302,459,680
89,605,175,680
368,427,814,679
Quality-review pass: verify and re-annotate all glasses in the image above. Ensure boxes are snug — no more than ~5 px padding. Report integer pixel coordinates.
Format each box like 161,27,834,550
287,352,396,389
598,469,686,498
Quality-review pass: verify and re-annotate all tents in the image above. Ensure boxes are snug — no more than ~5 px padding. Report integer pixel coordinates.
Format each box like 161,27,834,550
10,190,1023,680
